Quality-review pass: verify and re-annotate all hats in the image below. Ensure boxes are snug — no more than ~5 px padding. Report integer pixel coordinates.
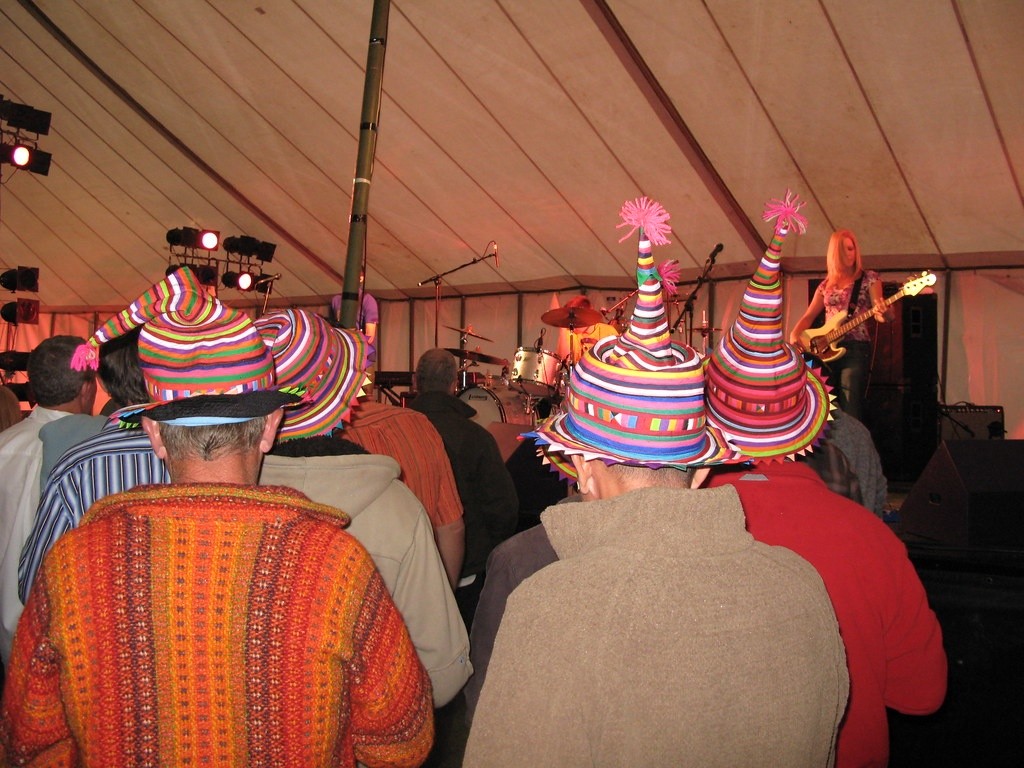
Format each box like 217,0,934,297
251,294,380,440
69,266,310,427
701,187,839,466
517,197,754,486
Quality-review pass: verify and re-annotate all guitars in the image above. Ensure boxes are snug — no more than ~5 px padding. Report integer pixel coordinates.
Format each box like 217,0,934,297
799,269,937,362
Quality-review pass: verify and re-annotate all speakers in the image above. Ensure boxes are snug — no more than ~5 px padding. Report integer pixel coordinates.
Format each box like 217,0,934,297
485,421,537,464
859,383,939,494
897,438,1024,551
938,404,1004,441
866,290,938,389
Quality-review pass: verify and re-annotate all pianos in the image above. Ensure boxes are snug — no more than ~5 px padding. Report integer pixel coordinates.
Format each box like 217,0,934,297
373,370,417,407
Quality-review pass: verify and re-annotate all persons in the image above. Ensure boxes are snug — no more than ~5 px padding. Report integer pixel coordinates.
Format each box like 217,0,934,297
255,305,474,706
459,198,852,768
0,267,433,768
705,190,946,768
404,349,519,592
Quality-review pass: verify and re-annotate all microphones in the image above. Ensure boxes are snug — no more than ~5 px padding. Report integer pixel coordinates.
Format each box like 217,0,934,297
538,329,544,346
964,425,976,438
494,242,500,268
706,244,724,264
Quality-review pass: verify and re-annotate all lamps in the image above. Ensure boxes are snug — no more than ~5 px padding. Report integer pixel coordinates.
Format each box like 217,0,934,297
1,97,52,176
0,266,39,293
0,298,40,325
166,226,277,297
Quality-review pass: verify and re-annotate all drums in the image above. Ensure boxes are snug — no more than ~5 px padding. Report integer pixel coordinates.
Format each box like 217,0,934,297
456,384,539,430
400,391,419,408
510,347,563,397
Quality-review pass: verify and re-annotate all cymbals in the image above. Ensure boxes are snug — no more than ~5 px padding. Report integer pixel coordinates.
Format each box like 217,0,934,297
540,306,604,328
441,325,494,343
446,347,511,366
686,327,723,332
